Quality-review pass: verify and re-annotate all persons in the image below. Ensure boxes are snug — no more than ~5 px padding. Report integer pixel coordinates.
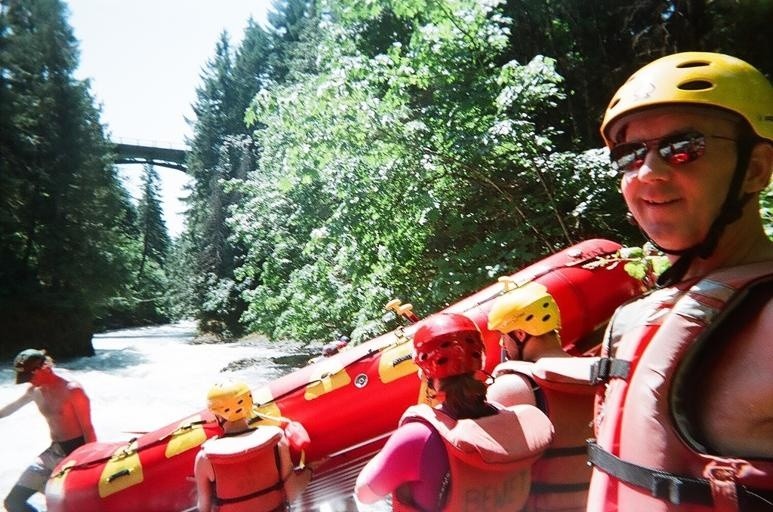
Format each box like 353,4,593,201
185,382,332,512
352,312,556,512
583,49,772,511
0,348,98,512
486,279,602,512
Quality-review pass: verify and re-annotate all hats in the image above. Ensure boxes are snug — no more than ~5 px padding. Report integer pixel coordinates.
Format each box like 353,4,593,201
13,348,46,384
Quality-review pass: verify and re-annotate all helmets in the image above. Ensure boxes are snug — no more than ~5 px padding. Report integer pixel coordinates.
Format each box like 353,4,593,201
207,384,253,422
413,313,487,379
486,281,563,337
599,51,773,142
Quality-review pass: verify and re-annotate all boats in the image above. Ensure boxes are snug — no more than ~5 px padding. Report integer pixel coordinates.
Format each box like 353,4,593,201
46,238,654,512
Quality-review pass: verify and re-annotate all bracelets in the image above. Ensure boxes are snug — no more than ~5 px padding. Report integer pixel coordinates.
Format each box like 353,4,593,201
302,465,315,474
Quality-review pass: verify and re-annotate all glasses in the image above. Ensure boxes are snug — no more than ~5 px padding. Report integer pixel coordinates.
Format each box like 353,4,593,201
609,130,740,172
